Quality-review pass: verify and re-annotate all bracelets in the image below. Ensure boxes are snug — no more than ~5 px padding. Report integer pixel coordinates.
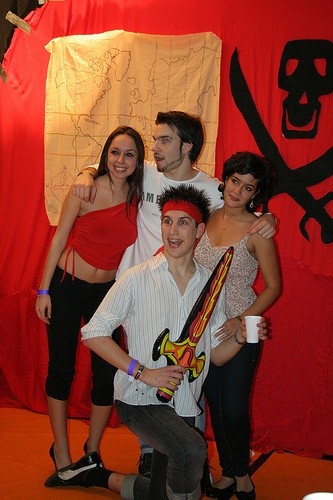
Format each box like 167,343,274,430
128,359,137,375
236,316,243,323
134,365,144,379
235,333,245,345
37,289,48,294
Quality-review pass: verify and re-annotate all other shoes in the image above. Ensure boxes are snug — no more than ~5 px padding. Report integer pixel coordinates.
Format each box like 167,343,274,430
235,473,255,500
208,476,236,499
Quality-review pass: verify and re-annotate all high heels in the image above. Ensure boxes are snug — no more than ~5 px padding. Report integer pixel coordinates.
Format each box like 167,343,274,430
83,437,88,455
49,442,57,471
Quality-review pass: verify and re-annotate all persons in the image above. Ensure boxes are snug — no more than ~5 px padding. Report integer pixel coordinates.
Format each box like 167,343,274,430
45,184,267,500
71,110,276,283
35,127,144,471
193,151,282,499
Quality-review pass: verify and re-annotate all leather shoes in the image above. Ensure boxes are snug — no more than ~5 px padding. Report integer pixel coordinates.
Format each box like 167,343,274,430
44,450,101,487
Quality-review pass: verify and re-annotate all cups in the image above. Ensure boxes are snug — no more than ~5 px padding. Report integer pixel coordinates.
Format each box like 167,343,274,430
244,315,262,343
302,492,333,500
